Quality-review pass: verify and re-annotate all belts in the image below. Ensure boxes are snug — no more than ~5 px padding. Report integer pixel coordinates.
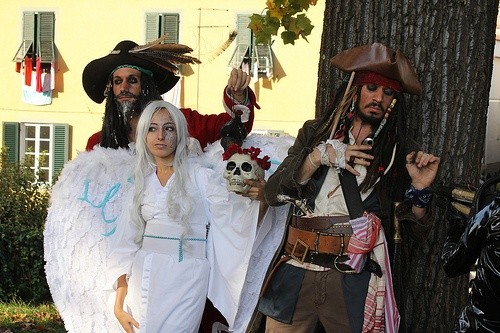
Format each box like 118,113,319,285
285,226,352,265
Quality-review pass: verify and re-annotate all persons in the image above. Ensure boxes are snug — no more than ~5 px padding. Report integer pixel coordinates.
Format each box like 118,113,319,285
257,42,440,333
83,32,260,333
440,161,500,333
110,101,275,333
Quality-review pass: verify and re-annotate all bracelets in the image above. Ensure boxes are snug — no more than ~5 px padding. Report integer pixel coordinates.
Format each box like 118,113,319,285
308,154,319,168
405,182,437,208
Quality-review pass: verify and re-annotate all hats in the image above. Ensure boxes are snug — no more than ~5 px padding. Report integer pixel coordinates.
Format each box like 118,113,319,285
330,43,423,139
81,35,201,104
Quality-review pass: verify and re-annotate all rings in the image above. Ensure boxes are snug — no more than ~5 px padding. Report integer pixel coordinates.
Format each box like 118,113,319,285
350,155,355,162
